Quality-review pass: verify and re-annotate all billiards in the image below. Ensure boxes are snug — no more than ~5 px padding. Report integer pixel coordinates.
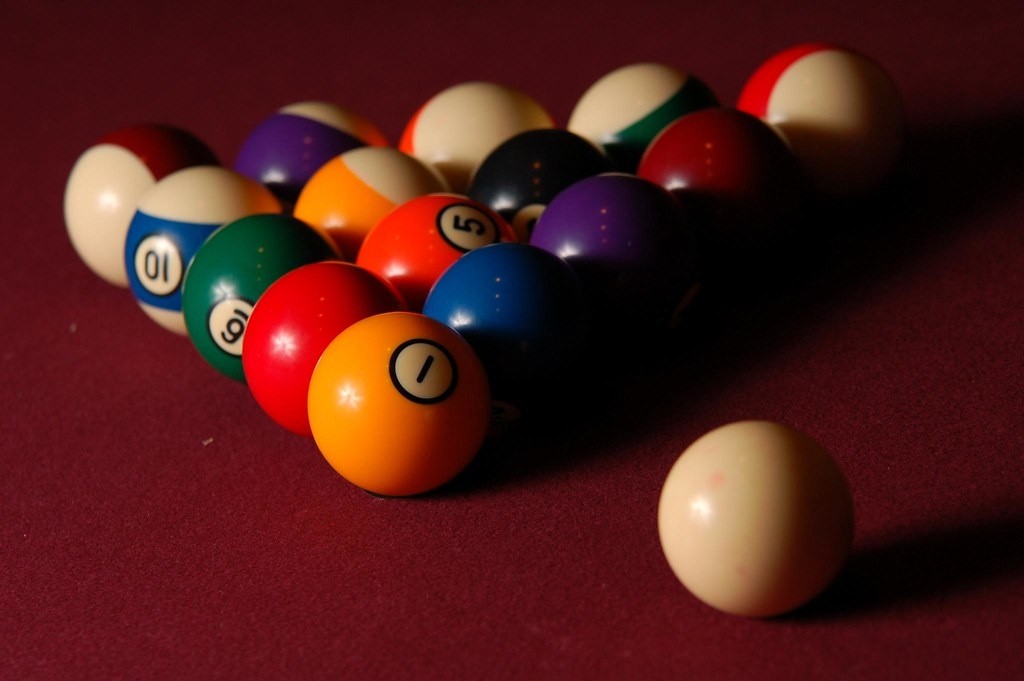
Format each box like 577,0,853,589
651,417,861,619
57,34,911,499
